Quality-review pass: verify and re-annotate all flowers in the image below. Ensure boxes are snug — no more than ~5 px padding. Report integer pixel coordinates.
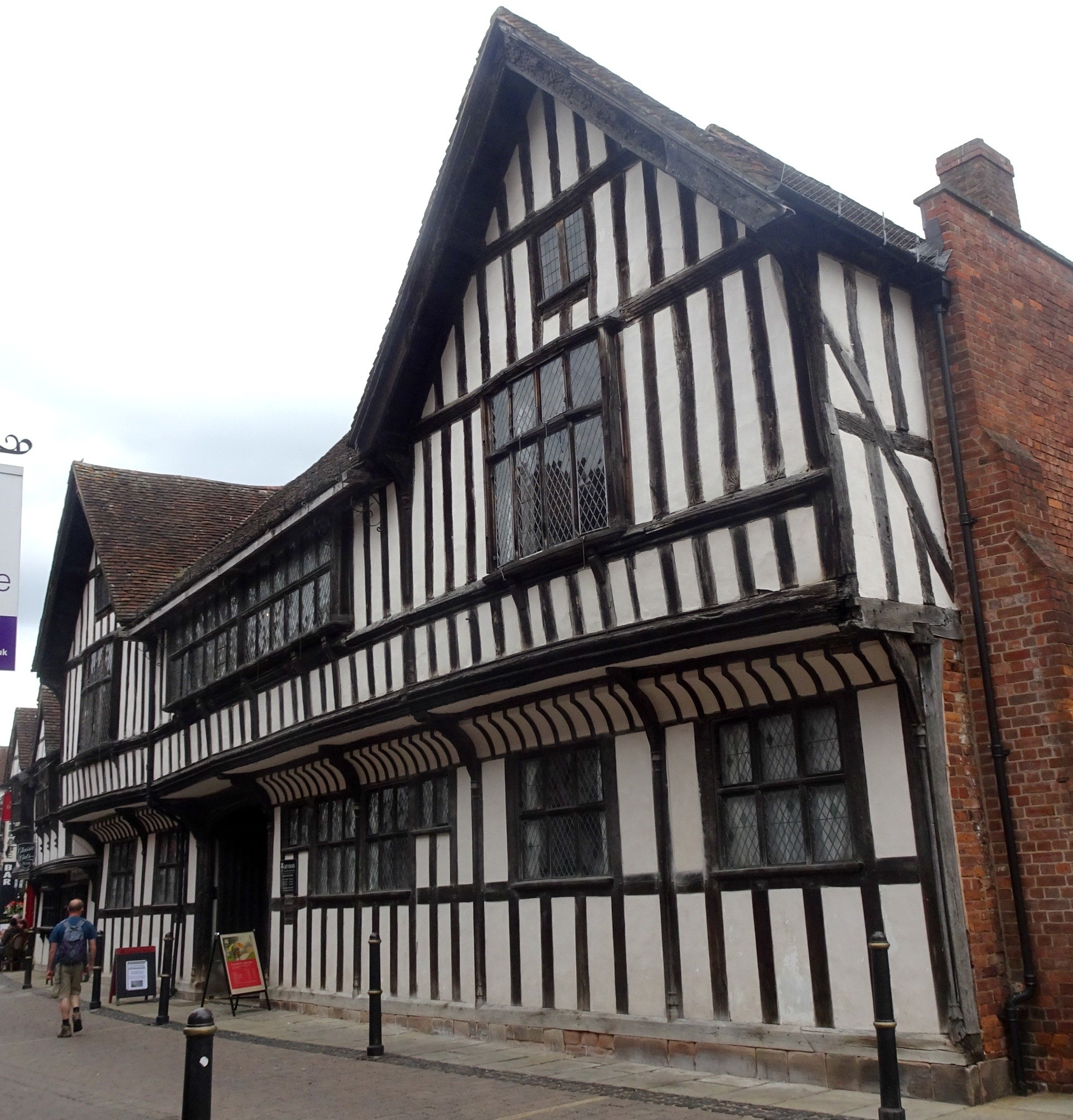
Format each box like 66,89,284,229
3,902,24,920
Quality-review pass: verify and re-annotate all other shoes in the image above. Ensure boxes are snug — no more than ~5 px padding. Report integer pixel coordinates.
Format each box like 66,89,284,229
72,1012,83,1032
57,1024,72,1037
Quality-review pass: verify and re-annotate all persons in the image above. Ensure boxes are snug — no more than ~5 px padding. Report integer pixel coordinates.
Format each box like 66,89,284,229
0,913,28,972
45,898,97,1038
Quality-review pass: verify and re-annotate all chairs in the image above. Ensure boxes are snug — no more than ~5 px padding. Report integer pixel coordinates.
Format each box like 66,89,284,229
0,931,29,973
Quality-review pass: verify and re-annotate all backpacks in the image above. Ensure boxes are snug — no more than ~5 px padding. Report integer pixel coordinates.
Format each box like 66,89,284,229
58,918,87,966
